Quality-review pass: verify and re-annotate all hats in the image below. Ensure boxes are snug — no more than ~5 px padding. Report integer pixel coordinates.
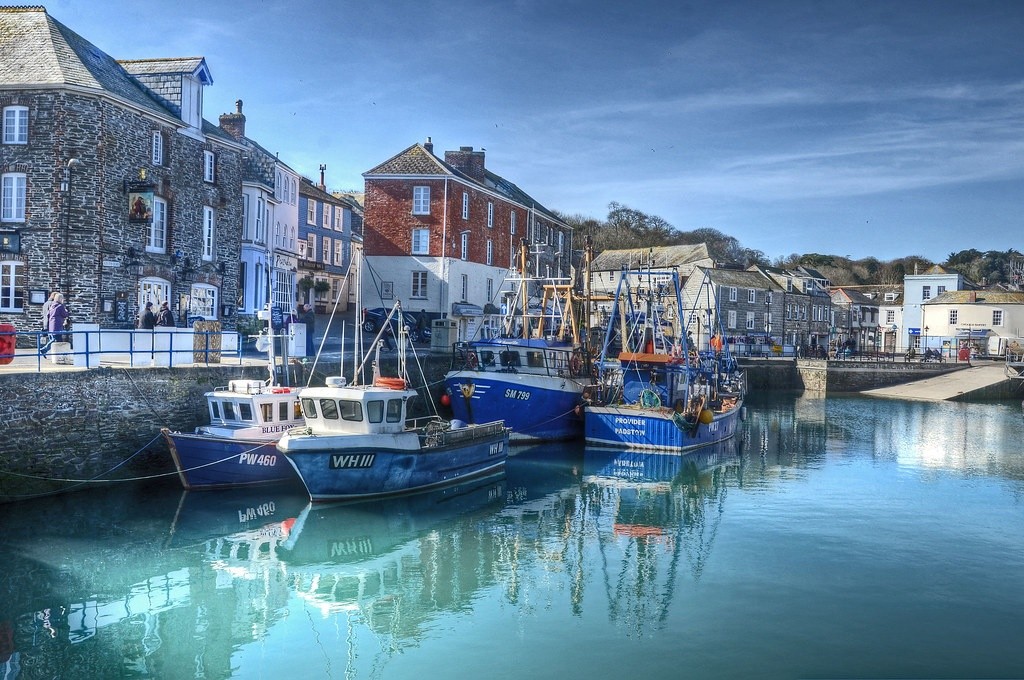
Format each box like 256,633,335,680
146,302,153,307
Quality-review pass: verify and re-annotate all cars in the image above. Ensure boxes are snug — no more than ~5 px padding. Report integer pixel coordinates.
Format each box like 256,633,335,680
364,308,432,343
634,311,673,326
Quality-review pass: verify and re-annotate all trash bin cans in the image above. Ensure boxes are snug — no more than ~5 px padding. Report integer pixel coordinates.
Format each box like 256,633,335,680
430,319,459,353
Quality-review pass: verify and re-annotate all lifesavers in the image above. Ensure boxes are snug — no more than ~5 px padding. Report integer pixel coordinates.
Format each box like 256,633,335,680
272,388,290,394
377,376,407,389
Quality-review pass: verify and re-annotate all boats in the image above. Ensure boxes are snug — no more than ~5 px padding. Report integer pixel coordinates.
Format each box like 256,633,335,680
276,470,508,570
275,246,514,505
162,480,309,553
158,252,410,492
442,234,749,453
583,436,741,631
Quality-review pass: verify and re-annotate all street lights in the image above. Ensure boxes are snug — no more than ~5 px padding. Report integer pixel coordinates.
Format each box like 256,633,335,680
876,324,882,361
765,286,774,345
795,322,800,359
924,326,929,347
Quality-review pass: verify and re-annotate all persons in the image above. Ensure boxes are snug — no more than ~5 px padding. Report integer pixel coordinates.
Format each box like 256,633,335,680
285,302,318,356
416,308,429,343
137,302,159,329
156,302,175,328
39,292,70,359
375,310,396,353
904,342,980,364
809,334,855,361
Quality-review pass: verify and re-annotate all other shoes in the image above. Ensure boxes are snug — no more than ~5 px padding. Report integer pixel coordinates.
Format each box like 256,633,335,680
40,348,48,359
